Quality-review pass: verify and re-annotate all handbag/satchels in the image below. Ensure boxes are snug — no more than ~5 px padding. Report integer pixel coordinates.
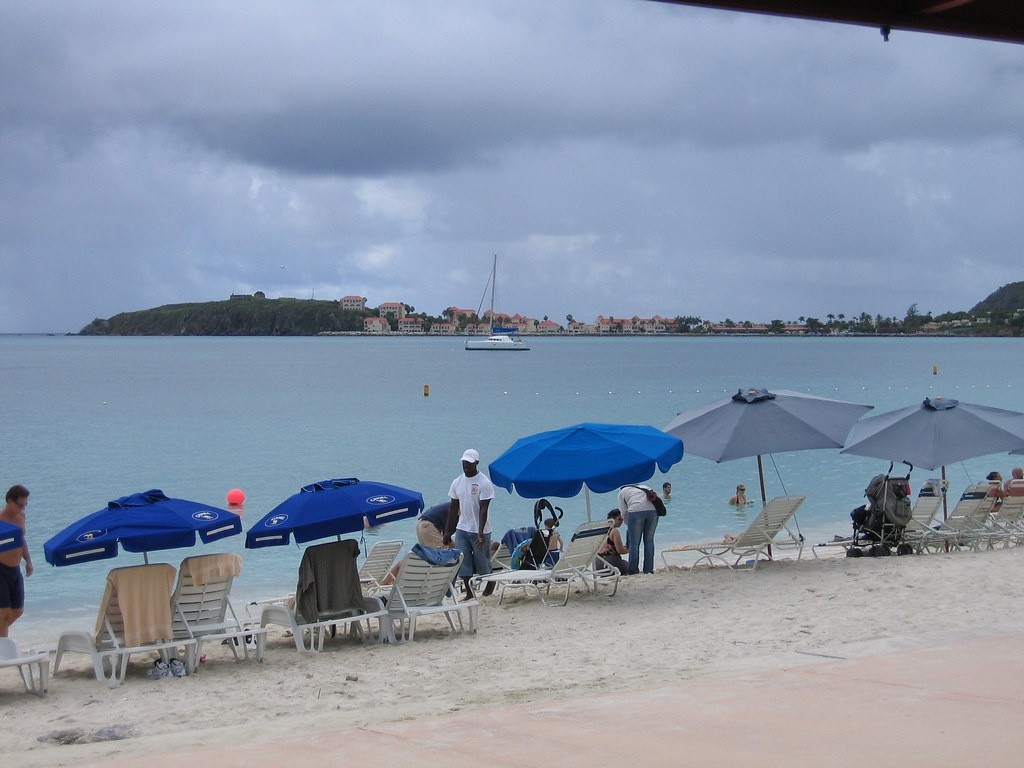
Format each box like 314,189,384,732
646,491,666,516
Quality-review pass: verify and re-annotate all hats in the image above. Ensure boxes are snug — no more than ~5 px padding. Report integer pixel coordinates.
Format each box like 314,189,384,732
460,449,479,463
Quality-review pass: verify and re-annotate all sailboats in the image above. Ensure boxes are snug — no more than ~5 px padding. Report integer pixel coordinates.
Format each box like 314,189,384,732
462,255,531,352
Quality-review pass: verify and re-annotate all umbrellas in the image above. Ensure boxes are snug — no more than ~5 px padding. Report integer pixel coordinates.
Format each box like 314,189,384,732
839,395,1024,553
244,477,425,552
659,383,874,556
487,422,685,589
43,489,243,565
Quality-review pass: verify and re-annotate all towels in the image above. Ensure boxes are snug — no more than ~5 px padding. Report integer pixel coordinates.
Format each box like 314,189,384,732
672,533,738,551
295,538,366,624
179,552,243,587
411,543,462,567
500,526,537,554
92,563,177,649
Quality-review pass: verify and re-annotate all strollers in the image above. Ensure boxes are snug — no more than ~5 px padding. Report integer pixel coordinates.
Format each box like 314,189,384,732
502,498,563,585
847,460,916,557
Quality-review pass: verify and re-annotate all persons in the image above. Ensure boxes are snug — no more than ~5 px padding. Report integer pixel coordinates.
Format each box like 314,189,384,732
662,482,672,497
415,501,460,600
0,484,34,638
985,472,1007,512
617,482,660,574
443,448,496,603
594,508,640,575
380,560,403,585
728,484,747,506
1003,468,1023,494
544,518,564,570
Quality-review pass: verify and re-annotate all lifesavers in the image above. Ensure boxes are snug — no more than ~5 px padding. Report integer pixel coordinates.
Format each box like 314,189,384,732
510,538,533,569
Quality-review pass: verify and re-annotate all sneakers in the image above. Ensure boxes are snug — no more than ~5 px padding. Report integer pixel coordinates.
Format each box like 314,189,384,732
147,658,173,679
168,657,186,676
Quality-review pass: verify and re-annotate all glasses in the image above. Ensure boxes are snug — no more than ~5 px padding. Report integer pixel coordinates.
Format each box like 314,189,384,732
11,497,28,509
739,489,745,491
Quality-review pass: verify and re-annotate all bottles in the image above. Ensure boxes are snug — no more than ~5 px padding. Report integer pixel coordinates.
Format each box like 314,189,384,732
20,648,43,691
896,482,906,497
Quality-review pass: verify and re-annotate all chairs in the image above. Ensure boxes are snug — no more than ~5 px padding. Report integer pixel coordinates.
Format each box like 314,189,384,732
660,495,805,572
468,518,623,607
287,540,404,597
488,526,537,570
813,478,1024,560
172,552,267,675
50,562,197,690
260,538,388,654
364,546,480,644
0,637,52,697
